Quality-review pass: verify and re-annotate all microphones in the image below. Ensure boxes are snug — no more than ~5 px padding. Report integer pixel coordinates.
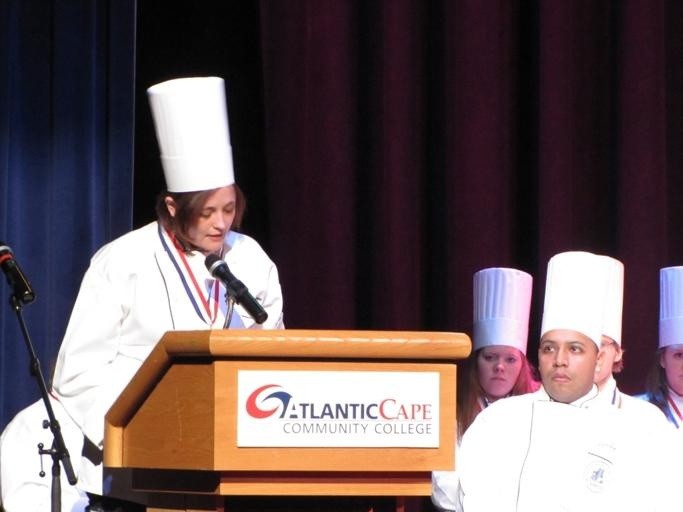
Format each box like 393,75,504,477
1,242,35,306
204,251,268,326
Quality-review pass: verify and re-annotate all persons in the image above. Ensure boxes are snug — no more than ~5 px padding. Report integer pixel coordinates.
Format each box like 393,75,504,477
595,336,676,446
634,345,683,511
0,396,105,511
428,347,542,512
52,183,291,512
455,329,664,512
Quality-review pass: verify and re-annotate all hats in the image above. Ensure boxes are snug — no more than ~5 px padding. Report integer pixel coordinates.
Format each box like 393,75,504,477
658,266,683,350
471,266,534,356
539,251,625,353
146,76,236,193
